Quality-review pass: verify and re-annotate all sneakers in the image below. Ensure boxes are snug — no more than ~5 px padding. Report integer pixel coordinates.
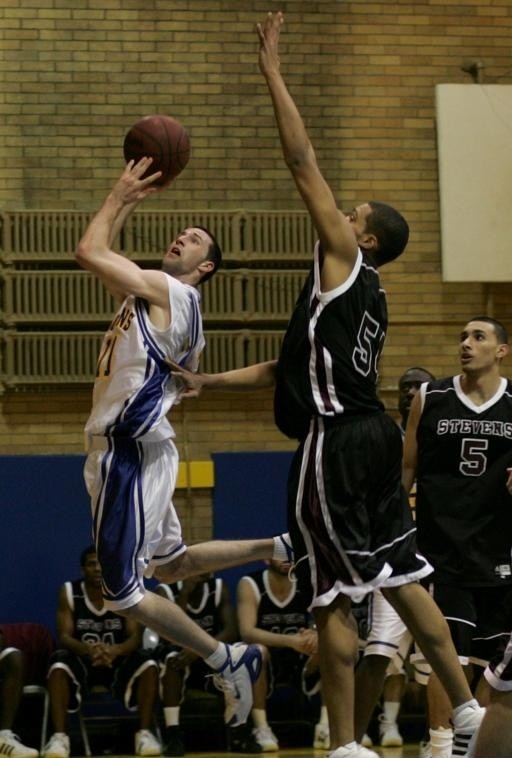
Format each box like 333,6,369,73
282,532,295,566
326,742,377,758
211,642,268,727
46,734,73,757
0,727,39,757
135,731,160,756
450,708,487,757
253,722,278,751
314,723,331,749
380,721,405,747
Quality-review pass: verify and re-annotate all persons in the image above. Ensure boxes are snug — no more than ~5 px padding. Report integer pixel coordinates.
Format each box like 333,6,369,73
402,316,512,756
161,7,491,755
355,367,437,748
73,155,298,730
0,543,512,756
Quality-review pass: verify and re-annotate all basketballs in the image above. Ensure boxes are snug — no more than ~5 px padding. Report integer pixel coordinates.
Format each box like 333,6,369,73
123,117,191,185
